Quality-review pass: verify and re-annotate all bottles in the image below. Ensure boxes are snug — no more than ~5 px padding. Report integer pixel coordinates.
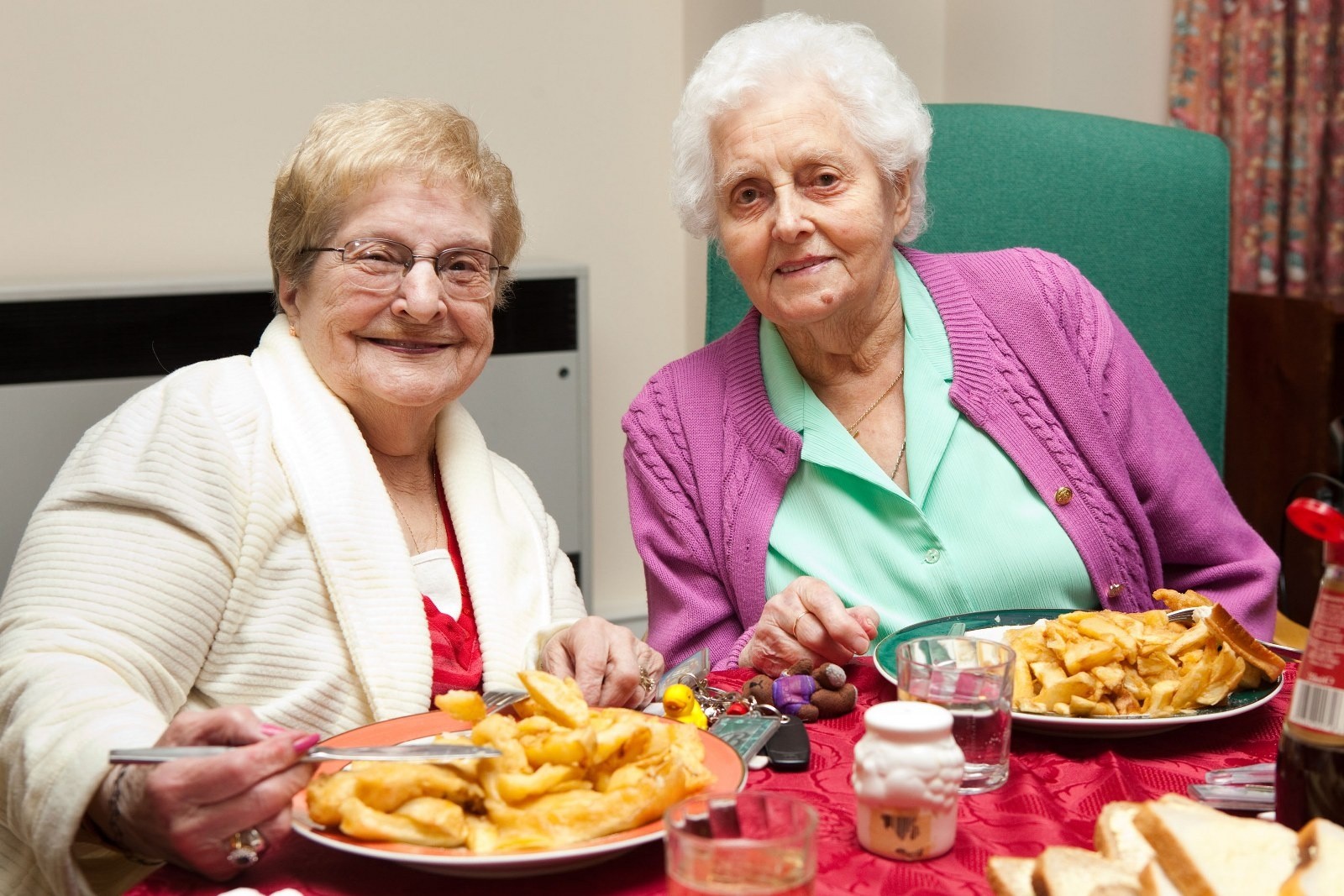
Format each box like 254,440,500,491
1273,474,1344,842
851,701,963,861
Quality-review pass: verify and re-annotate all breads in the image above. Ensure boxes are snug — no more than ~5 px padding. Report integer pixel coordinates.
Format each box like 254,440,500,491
986,792,1344,896
1192,603,1286,685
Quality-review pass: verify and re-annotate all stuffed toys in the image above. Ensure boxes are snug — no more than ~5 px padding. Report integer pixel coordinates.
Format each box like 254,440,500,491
739,663,859,723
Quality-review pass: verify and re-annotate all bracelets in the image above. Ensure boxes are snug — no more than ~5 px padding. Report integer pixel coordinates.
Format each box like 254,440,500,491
104,761,165,866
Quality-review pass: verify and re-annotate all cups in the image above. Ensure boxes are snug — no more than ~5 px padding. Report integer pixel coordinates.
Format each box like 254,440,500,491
663,791,820,896
898,634,1017,795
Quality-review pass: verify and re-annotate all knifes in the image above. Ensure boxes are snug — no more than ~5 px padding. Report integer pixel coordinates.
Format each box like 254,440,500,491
111,744,504,760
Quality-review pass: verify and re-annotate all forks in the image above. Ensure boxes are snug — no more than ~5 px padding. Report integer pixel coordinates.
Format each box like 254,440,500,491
477,688,529,716
1164,609,1304,661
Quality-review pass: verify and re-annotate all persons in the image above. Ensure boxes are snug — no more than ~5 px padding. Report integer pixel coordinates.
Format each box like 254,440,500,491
0,96,666,896
624,10,1282,680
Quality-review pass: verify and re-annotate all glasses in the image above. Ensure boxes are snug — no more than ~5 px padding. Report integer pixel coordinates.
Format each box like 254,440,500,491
296,238,509,300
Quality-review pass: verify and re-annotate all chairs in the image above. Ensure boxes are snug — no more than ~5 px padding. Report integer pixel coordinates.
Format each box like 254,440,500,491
706,103,1229,484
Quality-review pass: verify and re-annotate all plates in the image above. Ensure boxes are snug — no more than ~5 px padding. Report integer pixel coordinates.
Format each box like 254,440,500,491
873,608,1286,739
278,707,749,879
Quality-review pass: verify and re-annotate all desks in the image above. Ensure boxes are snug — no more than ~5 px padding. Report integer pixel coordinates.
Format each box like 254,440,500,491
115,656,1299,895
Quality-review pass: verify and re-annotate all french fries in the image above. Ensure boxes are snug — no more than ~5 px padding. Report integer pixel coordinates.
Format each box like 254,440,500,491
306,670,718,855
998,610,1261,715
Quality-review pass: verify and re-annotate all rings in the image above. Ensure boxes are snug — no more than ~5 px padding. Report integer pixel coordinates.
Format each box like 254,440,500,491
226,830,265,866
792,611,809,638
636,666,656,696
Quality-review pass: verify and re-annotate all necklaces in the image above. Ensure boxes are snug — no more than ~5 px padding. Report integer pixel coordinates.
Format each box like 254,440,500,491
889,439,909,484
376,459,440,554
845,369,903,439
